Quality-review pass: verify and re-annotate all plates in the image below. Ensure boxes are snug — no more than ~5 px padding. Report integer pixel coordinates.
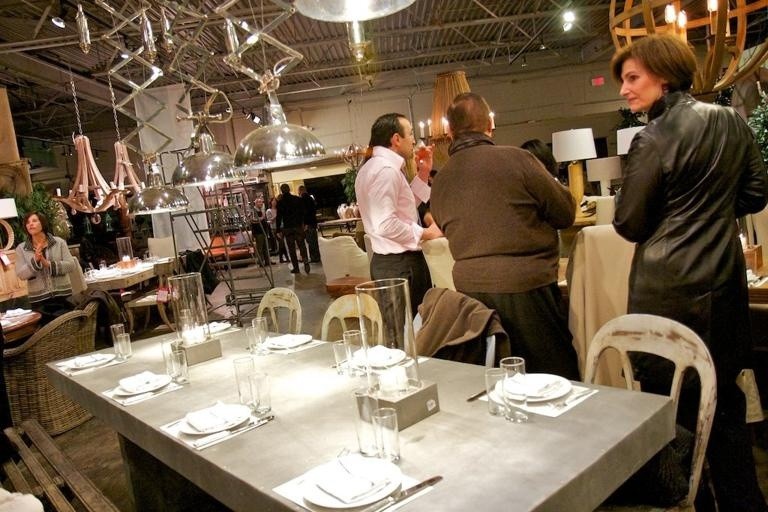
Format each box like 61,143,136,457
303,456,402,508
67,354,116,370
495,373,572,402
112,374,172,396
95,273,117,279
180,404,250,435
358,349,406,369
267,334,312,350
5,309,31,318
143,262,158,268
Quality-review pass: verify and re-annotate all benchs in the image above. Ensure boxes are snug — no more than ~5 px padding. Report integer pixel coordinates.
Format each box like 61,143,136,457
0,418,120,512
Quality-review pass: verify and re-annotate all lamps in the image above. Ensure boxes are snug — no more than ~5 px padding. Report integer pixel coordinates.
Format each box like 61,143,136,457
538,34,546,51
0,197,18,219
608,0,768,102
558,17,572,31
552,125,651,226
521,51,528,68
419,0,496,142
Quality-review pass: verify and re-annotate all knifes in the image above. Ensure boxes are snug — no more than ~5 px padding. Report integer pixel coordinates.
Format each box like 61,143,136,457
467,384,496,402
193,416,274,448
364,476,443,512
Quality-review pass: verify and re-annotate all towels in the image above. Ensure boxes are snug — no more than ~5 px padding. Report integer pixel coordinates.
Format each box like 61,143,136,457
315,448,390,504
504,371,554,398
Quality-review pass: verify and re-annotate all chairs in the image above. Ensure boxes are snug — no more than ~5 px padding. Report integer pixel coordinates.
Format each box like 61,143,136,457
404,301,496,367
582,313,717,511
565,226,637,386
419,237,455,293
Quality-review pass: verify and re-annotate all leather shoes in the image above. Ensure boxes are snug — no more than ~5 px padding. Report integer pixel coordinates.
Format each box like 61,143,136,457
265,262,276,265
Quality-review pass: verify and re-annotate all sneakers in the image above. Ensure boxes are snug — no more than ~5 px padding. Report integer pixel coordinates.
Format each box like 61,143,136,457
304,261,310,273
292,268,299,273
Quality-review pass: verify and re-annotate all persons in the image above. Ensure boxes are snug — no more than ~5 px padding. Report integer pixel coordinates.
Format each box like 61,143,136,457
266,198,290,263
353,113,445,351
298,185,320,263
519,139,559,178
14,210,76,329
427,170,437,186
131,216,149,260
419,200,434,227
610,35,768,510
277,184,310,274
428,91,579,383
249,199,276,266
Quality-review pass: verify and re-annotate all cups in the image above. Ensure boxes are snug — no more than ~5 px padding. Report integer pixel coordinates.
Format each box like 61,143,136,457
249,373,271,414
350,386,384,456
355,278,421,399
162,339,186,383
251,317,271,355
485,367,508,417
246,325,263,355
233,356,260,410
499,357,529,423
343,330,367,377
116,333,133,360
85,268,92,280
371,407,400,465
143,250,153,262
167,272,211,345
333,340,354,375
110,324,128,357
171,350,190,384
98,260,106,270
116,237,133,261
